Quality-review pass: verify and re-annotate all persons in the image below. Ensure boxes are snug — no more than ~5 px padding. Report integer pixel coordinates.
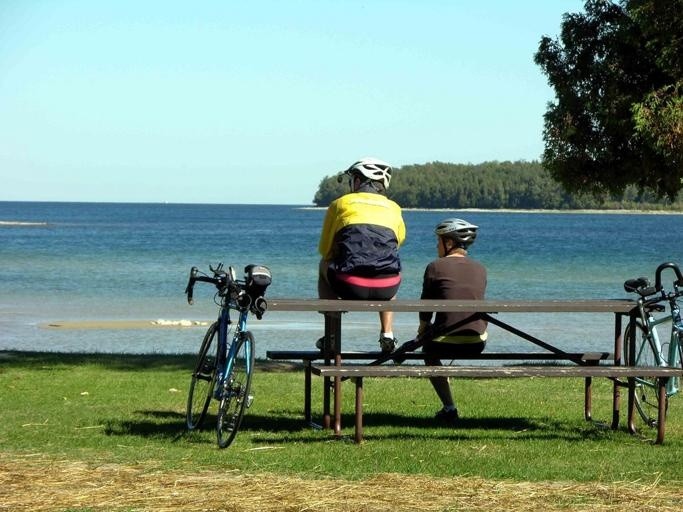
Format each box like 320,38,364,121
315,155,407,352
391,218,490,422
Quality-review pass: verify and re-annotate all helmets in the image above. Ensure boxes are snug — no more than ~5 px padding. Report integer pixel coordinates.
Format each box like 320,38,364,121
343,158,393,190
433,217,479,244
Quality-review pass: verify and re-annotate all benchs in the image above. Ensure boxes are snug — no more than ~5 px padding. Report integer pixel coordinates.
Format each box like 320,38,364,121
267,351,619,427
263,296,664,437
313,364,683,443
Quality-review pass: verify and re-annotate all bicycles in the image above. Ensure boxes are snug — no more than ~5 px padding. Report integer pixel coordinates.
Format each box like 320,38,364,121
623,262,683,429
183,262,268,448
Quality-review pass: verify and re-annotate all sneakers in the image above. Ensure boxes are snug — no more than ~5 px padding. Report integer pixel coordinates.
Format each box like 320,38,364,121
316,334,336,353
378,336,399,353
393,339,415,364
434,408,458,420
